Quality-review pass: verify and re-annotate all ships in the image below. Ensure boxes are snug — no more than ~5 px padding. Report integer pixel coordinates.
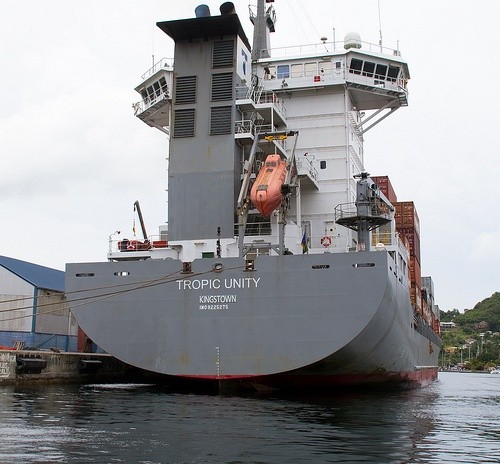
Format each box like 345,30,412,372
58,0,442,393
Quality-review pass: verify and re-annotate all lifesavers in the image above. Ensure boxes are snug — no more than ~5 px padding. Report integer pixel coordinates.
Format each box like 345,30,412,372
321,237,332,247
127,241,137,250
